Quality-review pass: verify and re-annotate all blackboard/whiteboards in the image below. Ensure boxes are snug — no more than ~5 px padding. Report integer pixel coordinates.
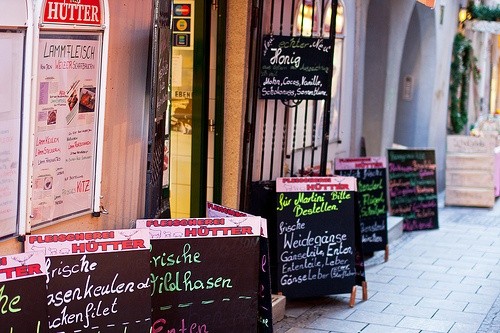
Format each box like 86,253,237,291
204,200,275,333
46,248,151,332
258,34,333,101
136,217,260,333
387,148,439,231
272,175,366,298
332,157,389,250
0,267,47,332
